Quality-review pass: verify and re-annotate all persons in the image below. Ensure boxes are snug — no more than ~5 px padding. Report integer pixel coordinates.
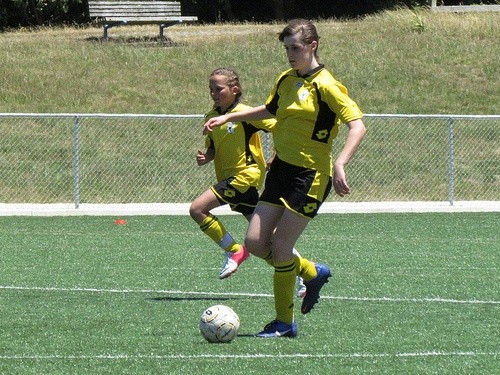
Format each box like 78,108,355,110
188,67,310,299
202,16,366,340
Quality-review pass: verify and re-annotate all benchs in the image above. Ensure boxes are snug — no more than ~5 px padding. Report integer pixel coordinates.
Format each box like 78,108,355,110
88,0,198,40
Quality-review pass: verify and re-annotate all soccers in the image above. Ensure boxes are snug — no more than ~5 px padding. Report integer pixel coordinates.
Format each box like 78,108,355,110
199,305,241,344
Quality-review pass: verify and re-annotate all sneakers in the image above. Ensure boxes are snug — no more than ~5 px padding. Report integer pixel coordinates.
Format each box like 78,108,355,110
219,244,250,278
254,318,298,339
295,276,307,298
300,264,332,315
